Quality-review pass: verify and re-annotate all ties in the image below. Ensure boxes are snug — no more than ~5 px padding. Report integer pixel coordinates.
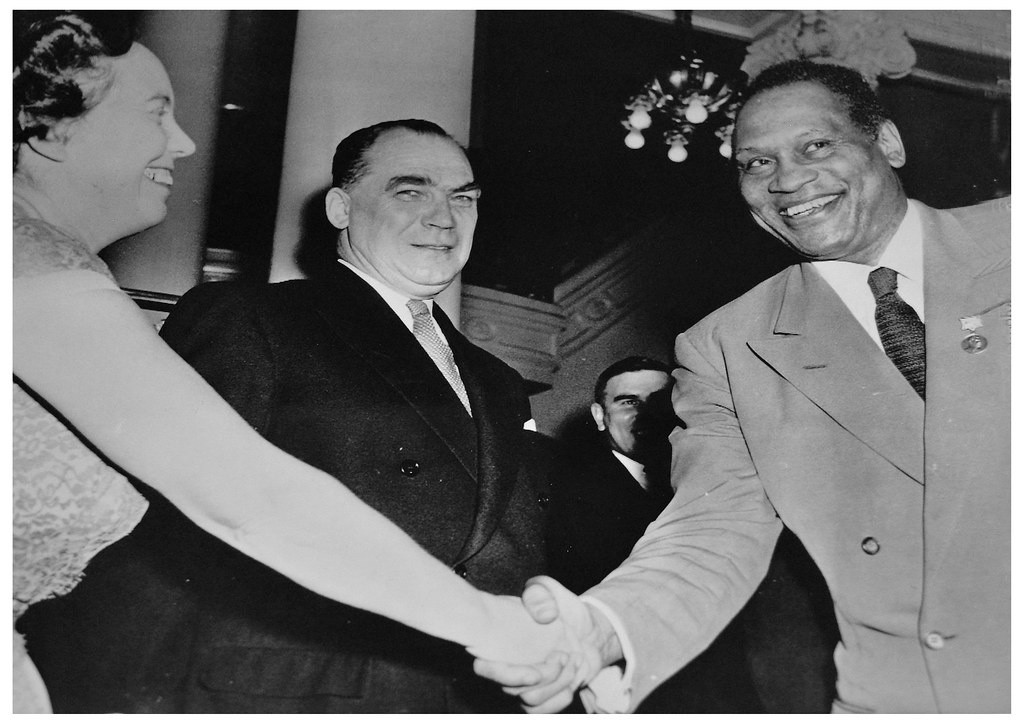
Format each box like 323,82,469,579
868,266,926,401
406,300,473,418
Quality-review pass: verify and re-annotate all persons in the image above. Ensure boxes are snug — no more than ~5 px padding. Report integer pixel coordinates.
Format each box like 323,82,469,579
472,58,1011,714
13,10,595,714
101,118,569,714
590,353,687,544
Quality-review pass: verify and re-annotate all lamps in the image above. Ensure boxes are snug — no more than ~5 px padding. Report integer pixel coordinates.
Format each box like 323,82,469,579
620,10,743,163
